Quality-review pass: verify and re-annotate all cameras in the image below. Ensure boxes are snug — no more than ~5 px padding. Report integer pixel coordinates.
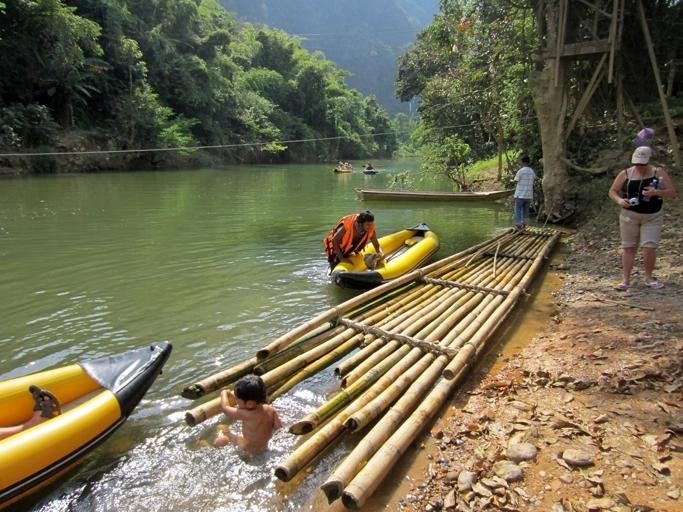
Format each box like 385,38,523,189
628,197,640,206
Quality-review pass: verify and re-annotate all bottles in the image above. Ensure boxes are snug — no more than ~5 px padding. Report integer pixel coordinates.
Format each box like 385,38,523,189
656,176,665,201
642,178,657,203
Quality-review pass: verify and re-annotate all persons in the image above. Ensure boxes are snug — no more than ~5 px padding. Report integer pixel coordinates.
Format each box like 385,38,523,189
336,160,352,169
213,373,282,453
0,385,62,440
367,163,373,170
607,146,676,291
510,155,537,233
323,209,385,272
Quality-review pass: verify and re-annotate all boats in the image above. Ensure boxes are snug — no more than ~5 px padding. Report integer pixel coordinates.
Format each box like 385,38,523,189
0,340,172,512
329,222,440,290
351,186,515,202
179,225,563,510
332,167,352,172
362,168,375,174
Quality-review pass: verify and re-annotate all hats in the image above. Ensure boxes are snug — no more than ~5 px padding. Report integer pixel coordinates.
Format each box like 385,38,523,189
359,210,374,222
632,146,652,164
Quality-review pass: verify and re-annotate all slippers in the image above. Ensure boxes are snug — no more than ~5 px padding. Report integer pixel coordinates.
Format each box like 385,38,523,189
613,283,631,291
645,280,665,289
29,385,61,418
